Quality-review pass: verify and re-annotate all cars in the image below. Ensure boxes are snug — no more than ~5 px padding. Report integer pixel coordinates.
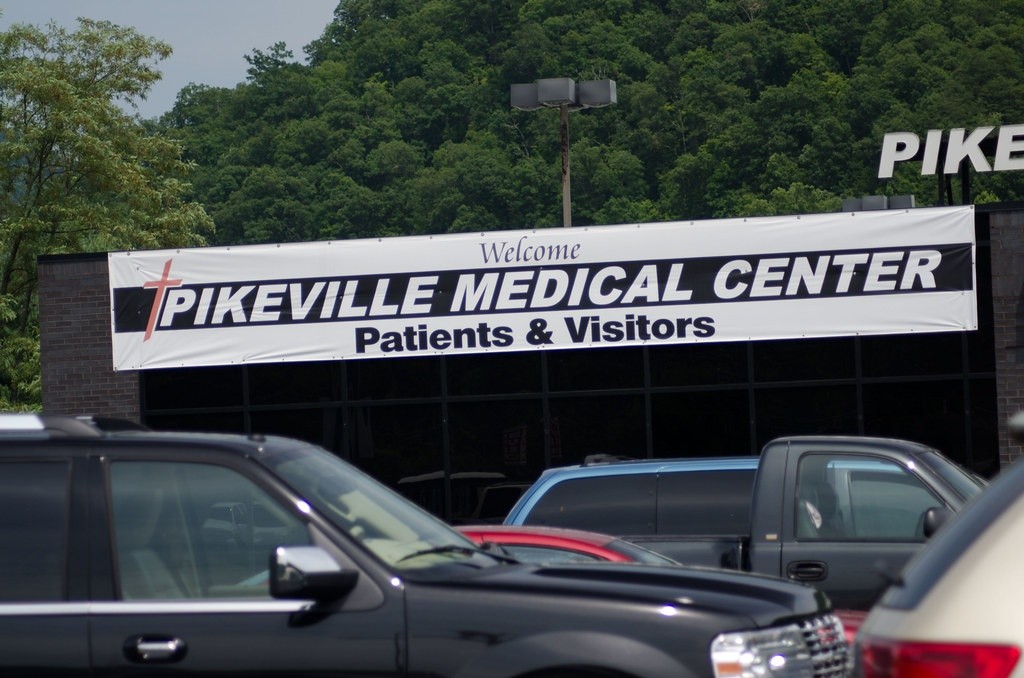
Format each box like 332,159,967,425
451,525,684,566
854,413,1022,678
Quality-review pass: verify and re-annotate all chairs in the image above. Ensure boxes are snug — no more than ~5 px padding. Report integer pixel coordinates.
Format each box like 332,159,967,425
109,461,193,604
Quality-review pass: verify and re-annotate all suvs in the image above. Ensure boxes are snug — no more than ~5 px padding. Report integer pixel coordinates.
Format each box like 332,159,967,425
0,414,849,677
499,454,995,540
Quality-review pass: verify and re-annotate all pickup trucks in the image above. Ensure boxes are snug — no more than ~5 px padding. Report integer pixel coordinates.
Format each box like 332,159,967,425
610,433,987,613
